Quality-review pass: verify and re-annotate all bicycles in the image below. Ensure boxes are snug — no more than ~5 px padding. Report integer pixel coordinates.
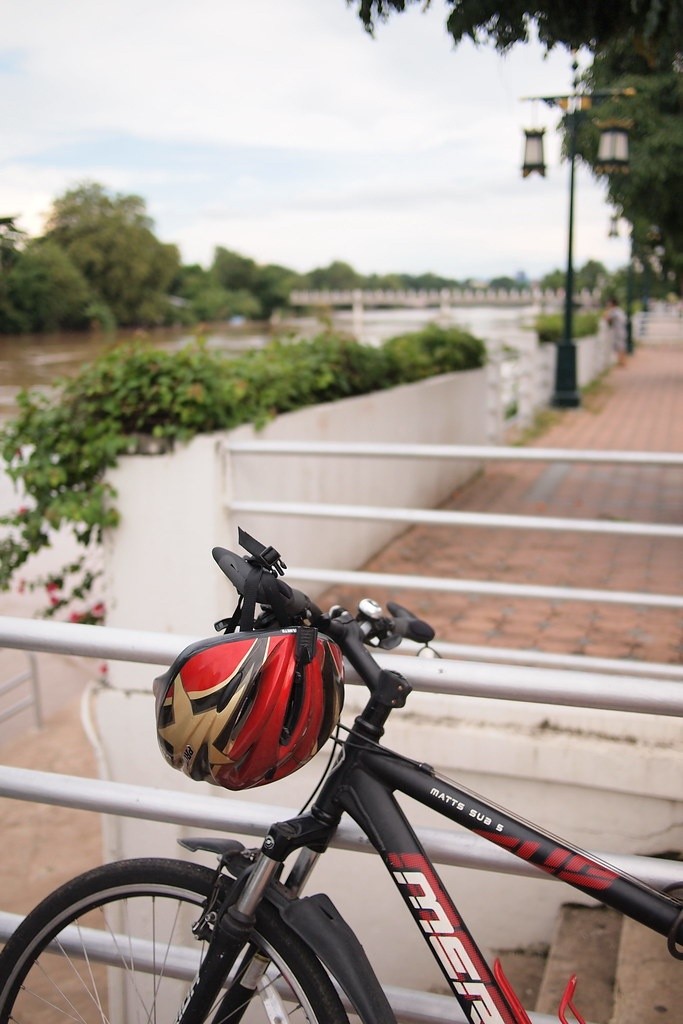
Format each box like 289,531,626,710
1,526,683,1024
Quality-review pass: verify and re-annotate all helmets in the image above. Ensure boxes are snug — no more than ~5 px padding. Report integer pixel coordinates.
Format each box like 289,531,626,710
152,625,345,791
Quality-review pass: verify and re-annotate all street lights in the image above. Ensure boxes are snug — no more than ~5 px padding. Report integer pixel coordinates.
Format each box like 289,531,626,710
517,83,636,405
